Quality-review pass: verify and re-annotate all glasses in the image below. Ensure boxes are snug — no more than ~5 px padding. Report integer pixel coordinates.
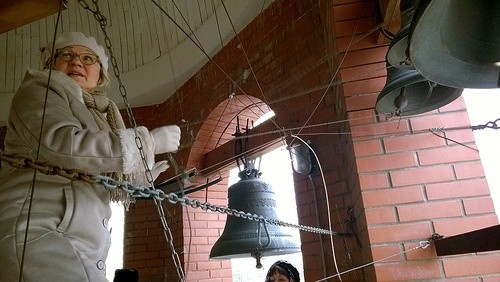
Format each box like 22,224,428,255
55,49,99,65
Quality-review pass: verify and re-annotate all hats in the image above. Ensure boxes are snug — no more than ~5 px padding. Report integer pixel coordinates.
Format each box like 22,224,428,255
43,32,109,75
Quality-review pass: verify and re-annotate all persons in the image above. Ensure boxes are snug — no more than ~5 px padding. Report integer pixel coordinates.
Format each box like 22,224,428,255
265,260,301,282
0,31,181,282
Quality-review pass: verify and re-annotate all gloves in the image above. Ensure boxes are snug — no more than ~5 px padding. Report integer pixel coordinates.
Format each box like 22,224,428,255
135,160,170,187
150,124,181,154
